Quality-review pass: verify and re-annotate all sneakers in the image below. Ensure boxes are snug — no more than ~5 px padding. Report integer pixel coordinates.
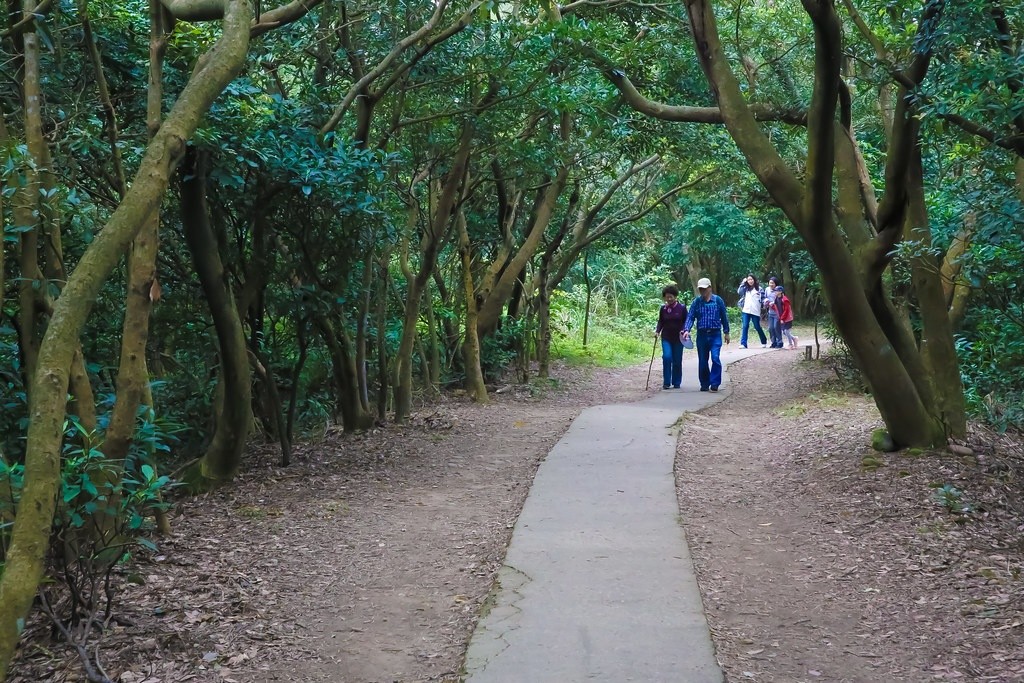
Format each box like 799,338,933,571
762,344,766,347
700,387,709,391
770,342,777,347
776,342,783,348
739,345,746,349
793,336,798,347
710,387,718,392
784,344,793,350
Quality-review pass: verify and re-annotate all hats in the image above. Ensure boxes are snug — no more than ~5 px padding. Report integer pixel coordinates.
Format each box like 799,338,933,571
697,278,711,289
679,330,693,350
773,286,784,293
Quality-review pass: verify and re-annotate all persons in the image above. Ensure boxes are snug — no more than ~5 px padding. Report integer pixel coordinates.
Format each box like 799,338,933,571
769,286,800,352
654,286,690,392
764,276,786,349
738,273,769,350
684,278,731,395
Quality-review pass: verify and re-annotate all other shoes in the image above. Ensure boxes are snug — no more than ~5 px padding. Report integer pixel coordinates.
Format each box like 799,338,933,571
674,386,680,388
663,385,669,389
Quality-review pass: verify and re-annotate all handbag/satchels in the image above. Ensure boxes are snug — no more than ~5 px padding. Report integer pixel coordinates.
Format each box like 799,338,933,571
738,297,744,308
